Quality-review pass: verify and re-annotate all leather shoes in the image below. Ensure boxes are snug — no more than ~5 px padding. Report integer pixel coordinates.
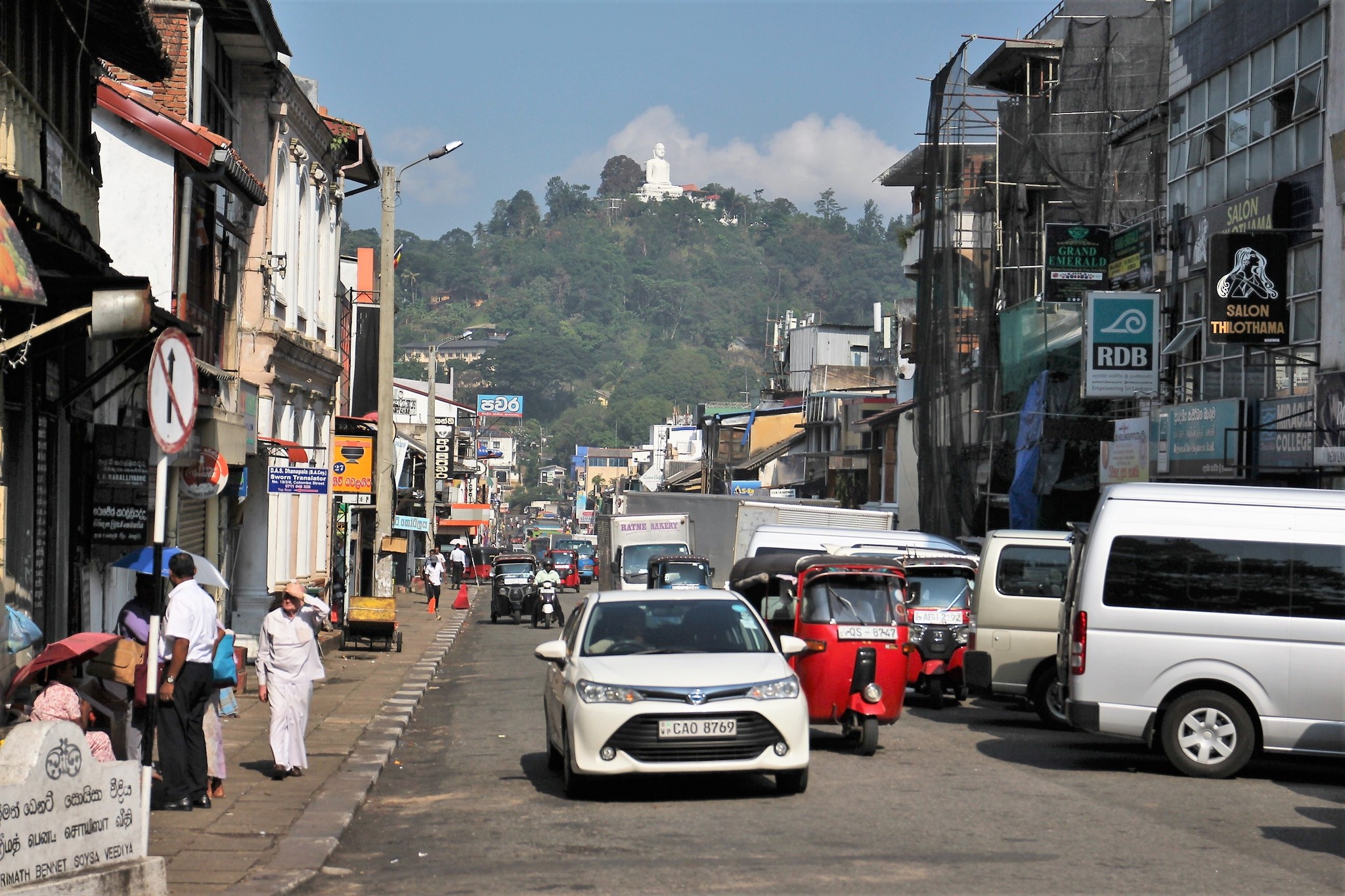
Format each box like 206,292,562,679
151,796,193,811
191,792,212,809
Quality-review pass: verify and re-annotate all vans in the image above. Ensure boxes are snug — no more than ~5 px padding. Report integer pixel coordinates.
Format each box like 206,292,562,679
960,522,1082,734
1045,480,1345,783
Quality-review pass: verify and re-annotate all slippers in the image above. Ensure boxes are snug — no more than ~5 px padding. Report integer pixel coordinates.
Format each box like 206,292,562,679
209,793,229,800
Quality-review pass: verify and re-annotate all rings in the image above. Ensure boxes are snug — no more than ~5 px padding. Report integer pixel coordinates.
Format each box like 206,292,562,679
166,697,169,699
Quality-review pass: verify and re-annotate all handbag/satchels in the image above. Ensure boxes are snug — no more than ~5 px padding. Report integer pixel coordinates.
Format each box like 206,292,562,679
84,602,152,687
4,605,44,656
134,630,168,708
212,626,238,689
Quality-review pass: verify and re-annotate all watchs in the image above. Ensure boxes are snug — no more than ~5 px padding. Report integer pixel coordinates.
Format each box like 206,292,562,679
165,676,175,684
302,593,306,600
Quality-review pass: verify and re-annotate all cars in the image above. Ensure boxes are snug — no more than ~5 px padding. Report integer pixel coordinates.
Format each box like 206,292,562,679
531,586,813,797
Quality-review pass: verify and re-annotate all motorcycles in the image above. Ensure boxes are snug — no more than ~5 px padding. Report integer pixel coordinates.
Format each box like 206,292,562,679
451,514,599,626
725,551,922,756
818,542,981,710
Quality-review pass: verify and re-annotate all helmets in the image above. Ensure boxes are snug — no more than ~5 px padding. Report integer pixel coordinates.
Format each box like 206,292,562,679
542,557,554,567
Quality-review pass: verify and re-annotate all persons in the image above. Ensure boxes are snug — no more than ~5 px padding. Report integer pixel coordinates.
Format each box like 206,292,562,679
563,516,573,524
515,516,535,530
530,557,565,627
511,522,514,530
811,580,877,623
462,545,466,547
510,517,513,523
255,582,330,779
208,594,226,797
450,544,466,590
30,658,117,761
117,571,163,786
424,546,447,613
589,606,661,655
151,553,217,811
566,524,571,533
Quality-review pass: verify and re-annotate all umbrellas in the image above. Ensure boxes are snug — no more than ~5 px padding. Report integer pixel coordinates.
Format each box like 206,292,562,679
7,632,126,727
109,546,230,602
450,539,468,545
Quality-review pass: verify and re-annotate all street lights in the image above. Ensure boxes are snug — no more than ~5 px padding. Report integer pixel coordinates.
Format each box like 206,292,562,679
425,331,475,559
369,138,466,597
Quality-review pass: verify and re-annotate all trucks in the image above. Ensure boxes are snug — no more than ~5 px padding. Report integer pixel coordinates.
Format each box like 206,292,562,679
597,510,695,593
621,488,980,595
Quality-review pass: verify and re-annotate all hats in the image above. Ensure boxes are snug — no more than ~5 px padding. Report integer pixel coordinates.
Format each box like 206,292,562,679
430,555,438,563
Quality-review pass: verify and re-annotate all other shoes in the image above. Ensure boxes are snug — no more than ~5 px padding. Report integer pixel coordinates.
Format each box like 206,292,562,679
272,764,284,778
451,585,454,590
286,765,302,777
558,621,565,627
528,623,537,629
457,586,460,590
435,607,438,611
